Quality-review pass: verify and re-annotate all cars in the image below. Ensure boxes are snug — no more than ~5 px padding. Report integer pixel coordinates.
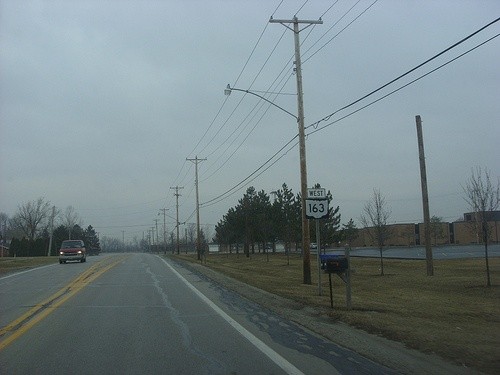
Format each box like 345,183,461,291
228,240,330,252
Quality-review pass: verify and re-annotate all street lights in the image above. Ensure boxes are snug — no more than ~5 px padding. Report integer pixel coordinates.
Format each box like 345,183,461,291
157,185,184,254
223,17,324,286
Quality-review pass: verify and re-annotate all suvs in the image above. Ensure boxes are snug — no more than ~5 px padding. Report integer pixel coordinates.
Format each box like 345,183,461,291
59,240,87,263
